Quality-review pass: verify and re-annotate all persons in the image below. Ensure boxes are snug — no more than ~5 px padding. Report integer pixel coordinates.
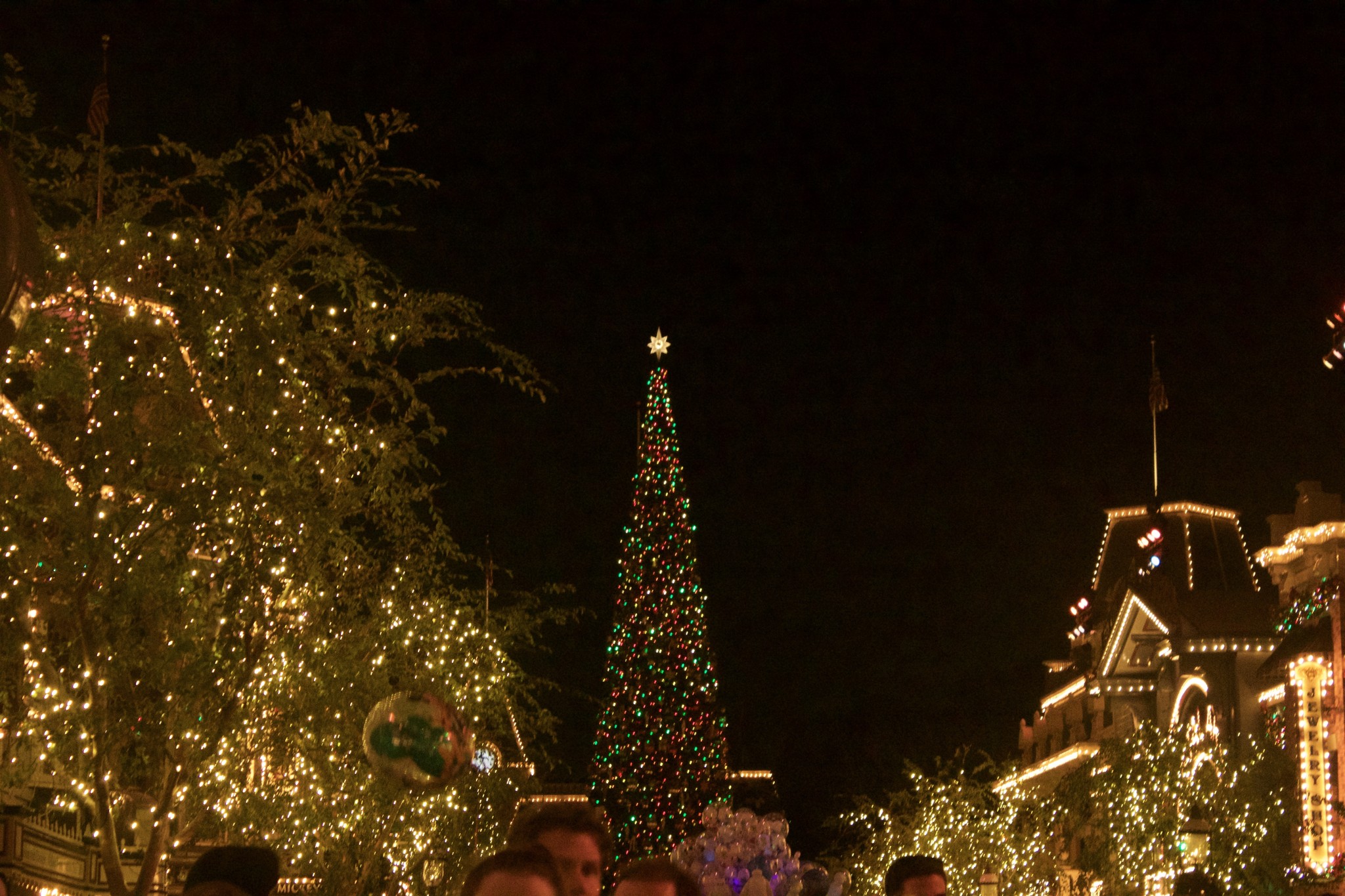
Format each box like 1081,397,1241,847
518,799,612,896
461,850,568,895
797,869,829,896
612,860,704,896
1171,870,1223,896
884,854,947,896
